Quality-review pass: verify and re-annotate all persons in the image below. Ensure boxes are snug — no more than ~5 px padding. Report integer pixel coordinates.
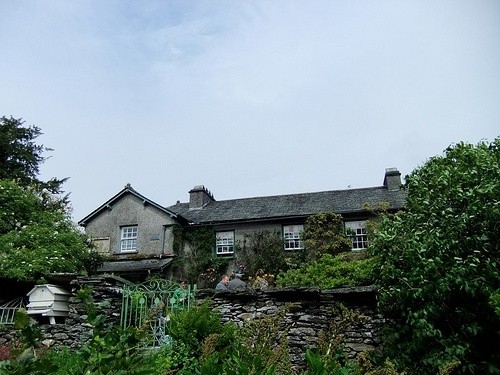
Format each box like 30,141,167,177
214,274,229,294
226,268,246,295
173,280,190,311
251,269,269,290
